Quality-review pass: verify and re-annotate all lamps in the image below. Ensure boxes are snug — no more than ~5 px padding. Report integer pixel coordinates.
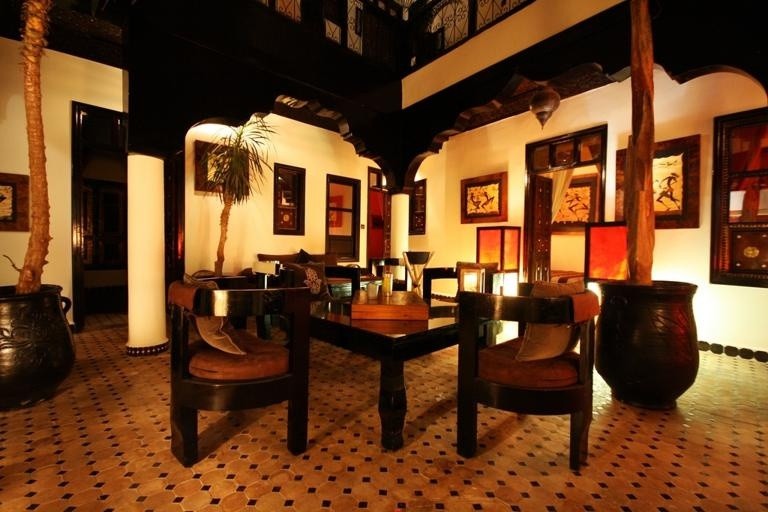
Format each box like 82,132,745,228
584,220,630,281
475,226,521,295
528,86,561,131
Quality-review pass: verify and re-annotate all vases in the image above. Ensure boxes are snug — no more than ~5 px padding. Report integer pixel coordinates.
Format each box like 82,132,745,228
0,284,77,411
594,280,699,412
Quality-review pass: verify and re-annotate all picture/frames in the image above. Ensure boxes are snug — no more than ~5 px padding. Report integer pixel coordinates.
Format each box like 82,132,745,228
550,172,601,235
273,162,306,236
614,134,701,230
193,139,250,196
0,173,30,233
460,171,508,224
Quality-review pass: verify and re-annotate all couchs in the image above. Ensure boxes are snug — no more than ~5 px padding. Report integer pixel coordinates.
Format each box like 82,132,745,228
236,252,361,283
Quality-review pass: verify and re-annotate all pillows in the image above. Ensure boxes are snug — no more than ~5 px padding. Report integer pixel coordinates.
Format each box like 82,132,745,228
294,248,316,264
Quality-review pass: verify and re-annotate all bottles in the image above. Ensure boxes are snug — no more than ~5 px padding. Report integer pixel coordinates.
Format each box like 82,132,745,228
357,264,395,305
